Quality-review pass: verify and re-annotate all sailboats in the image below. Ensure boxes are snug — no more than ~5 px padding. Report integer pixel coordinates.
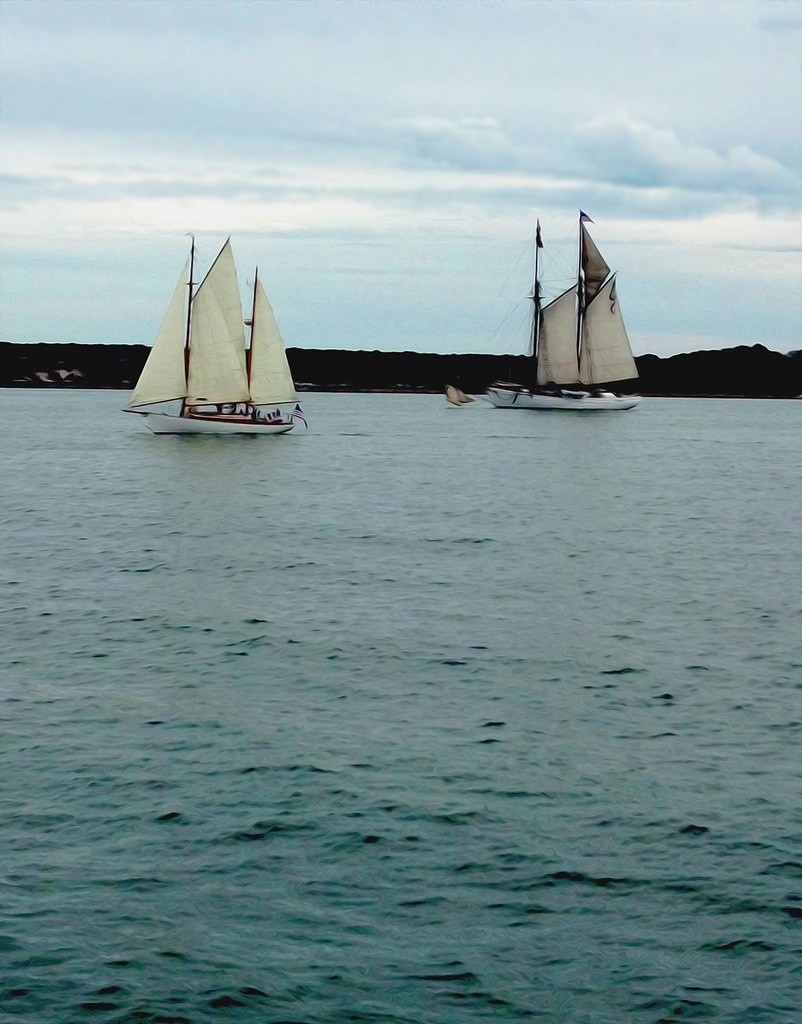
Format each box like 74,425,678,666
484,208,645,412
121,229,302,434
